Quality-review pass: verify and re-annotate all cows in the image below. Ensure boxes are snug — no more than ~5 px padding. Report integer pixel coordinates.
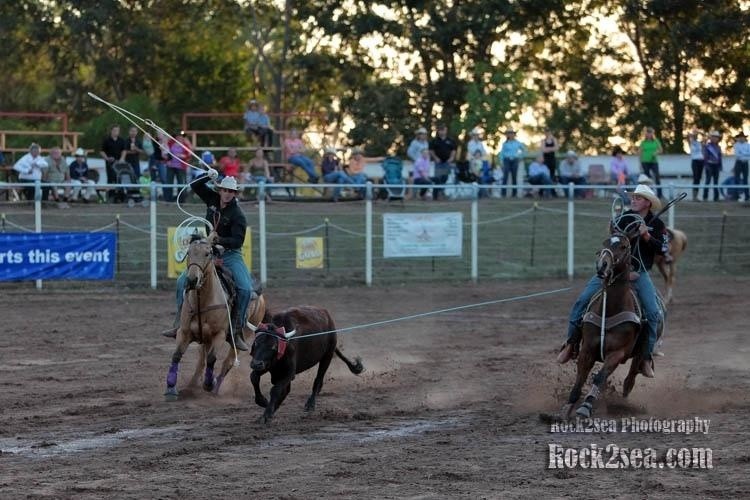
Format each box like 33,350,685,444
245,306,367,425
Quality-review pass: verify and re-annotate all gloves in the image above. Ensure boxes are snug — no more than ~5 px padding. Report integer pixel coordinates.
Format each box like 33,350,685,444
207,168,219,180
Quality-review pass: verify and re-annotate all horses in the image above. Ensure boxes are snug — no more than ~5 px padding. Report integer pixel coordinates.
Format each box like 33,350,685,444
653,226,688,304
554,219,674,431
163,226,273,402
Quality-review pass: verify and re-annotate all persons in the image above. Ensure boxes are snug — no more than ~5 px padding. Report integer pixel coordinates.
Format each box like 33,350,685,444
161,168,252,352
555,183,667,380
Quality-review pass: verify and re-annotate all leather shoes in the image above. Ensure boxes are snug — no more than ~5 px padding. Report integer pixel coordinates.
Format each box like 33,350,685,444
228,333,249,352
160,327,178,339
640,360,655,379
557,342,576,364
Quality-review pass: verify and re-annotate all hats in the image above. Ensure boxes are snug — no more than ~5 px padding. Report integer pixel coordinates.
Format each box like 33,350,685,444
326,148,337,156
710,130,723,142
213,175,240,193
735,131,748,138
468,128,484,135
506,129,517,133
612,147,629,156
72,148,87,157
415,127,428,135
566,150,576,158
624,184,663,212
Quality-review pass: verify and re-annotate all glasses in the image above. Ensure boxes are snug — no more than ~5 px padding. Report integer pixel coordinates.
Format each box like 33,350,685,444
220,188,234,193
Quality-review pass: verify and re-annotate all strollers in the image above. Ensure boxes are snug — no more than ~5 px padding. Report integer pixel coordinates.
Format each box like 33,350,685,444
378,156,408,207
436,159,486,200
111,161,152,209
